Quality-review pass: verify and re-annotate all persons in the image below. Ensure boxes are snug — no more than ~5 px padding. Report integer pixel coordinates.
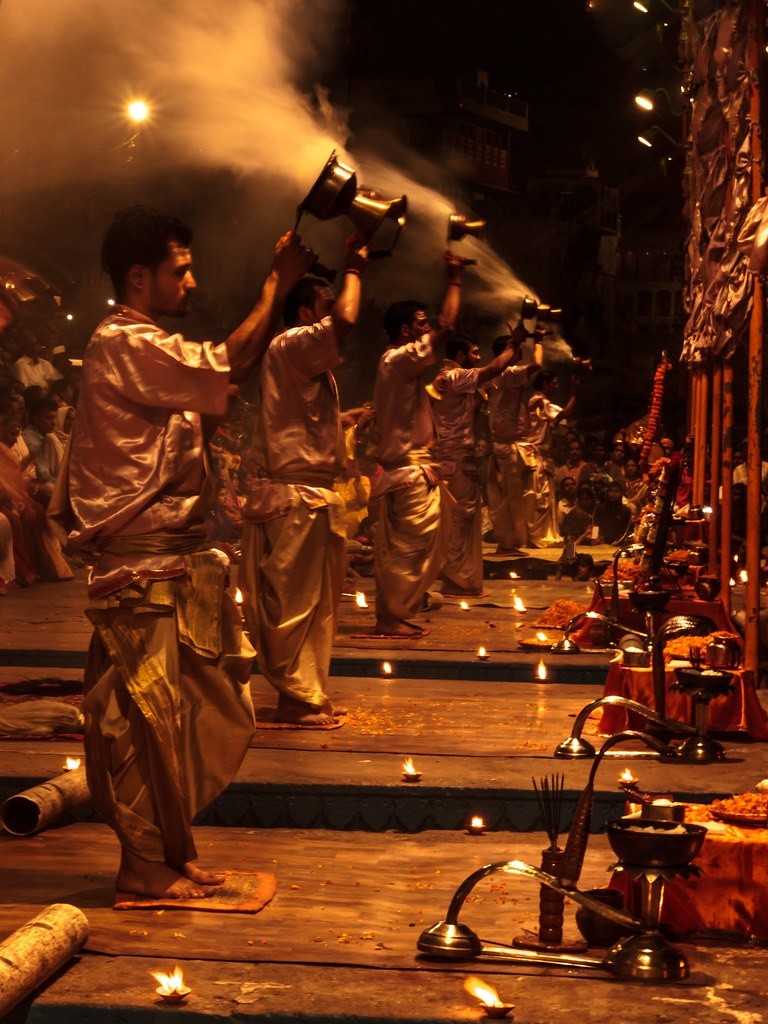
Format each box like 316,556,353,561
555,422,768,557
486,318,546,555
468,439,504,542
0,327,99,596
432,334,522,595
206,383,255,564
515,369,581,547
236,245,374,726
44,207,306,898
331,399,375,579
373,255,467,636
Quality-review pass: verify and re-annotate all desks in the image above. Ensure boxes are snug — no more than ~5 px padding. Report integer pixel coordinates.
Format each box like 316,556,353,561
572,592,731,647
608,803,768,945
597,658,768,740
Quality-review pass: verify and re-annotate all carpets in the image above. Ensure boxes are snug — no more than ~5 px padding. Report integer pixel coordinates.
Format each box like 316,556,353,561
114,869,278,913
